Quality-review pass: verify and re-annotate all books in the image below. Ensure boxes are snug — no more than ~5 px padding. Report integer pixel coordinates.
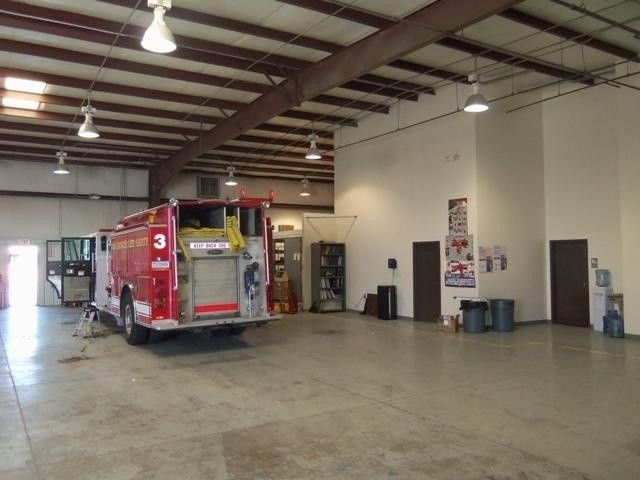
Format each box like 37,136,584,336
319,246,344,302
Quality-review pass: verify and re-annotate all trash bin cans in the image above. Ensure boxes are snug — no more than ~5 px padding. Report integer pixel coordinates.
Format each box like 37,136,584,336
461,298,514,334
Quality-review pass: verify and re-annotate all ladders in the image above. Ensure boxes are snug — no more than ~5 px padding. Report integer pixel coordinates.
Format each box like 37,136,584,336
72,305,104,338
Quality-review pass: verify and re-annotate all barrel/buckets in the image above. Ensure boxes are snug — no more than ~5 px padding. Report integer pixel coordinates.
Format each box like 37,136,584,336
608,310,624,337
489,299,514,332
604,310,613,334
596,269,610,286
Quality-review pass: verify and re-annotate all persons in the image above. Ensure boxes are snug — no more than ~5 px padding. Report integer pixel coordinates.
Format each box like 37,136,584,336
453,199,466,212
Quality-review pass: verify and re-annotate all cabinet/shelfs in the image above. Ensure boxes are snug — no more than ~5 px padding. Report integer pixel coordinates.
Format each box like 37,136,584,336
309,240,347,313
274,239,285,276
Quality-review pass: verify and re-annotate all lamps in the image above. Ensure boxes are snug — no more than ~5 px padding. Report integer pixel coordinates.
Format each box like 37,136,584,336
463,54,492,115
222,119,325,186
54,0,178,176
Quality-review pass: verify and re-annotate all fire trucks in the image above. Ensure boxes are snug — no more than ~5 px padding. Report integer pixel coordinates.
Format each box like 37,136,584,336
43,189,284,346
